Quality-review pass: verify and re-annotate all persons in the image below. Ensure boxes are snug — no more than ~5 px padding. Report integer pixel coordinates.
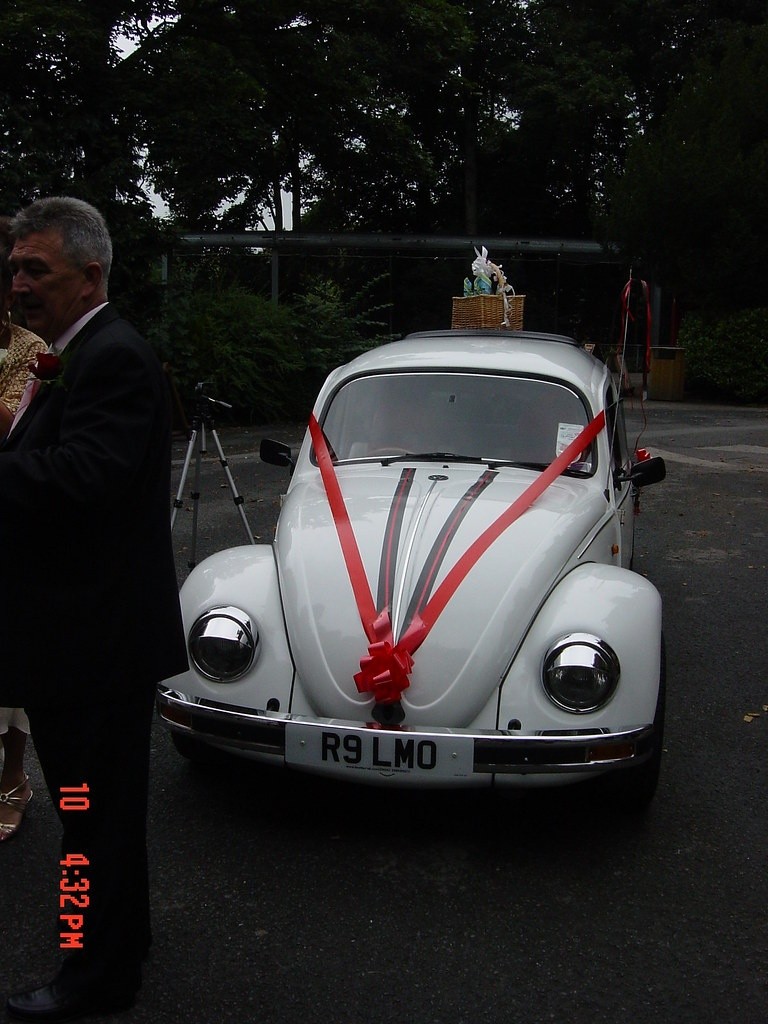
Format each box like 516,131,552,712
0,198,189,1023
0,215,50,843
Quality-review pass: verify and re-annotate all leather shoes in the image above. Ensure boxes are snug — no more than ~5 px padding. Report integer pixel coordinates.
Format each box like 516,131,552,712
6,946,150,1024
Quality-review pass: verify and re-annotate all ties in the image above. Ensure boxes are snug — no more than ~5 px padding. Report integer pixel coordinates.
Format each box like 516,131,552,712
7,360,42,441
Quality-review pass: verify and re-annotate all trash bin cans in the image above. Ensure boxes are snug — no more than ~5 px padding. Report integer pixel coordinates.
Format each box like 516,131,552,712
646,346,685,401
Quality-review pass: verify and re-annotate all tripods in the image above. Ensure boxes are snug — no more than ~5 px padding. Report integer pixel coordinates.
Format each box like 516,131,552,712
171,395,256,572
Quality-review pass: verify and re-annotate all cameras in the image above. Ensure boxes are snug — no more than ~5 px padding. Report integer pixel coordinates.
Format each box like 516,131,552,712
195,382,216,396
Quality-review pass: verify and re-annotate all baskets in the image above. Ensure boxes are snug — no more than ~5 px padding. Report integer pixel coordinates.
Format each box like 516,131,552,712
451,260,526,332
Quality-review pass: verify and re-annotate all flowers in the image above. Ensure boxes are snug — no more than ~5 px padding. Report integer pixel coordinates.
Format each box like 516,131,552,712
18,342,83,399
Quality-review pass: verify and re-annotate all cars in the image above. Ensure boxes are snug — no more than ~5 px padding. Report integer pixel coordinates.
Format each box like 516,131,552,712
154,328,664,838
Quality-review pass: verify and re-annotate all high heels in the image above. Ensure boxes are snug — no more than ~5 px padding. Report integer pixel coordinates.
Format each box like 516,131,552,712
0,771,34,842
